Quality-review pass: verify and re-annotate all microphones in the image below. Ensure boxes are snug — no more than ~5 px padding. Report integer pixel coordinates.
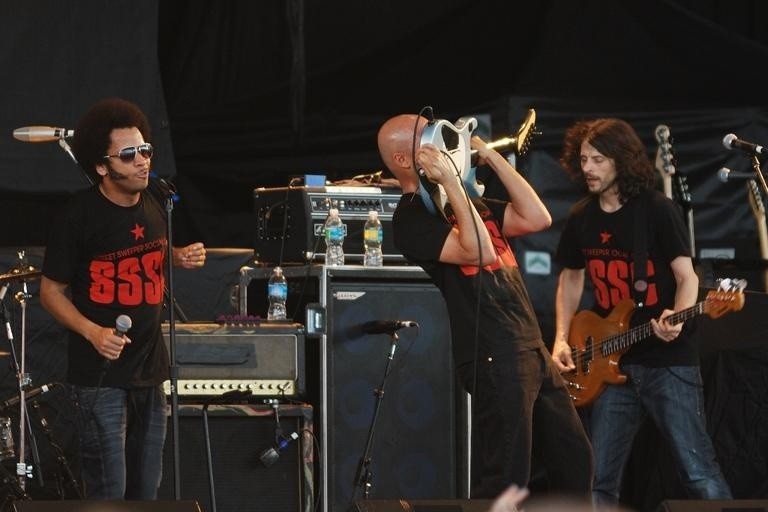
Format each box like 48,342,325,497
0,385,54,416
12,127,74,142
260,433,298,468
717,167,759,183
723,133,764,156
98,314,133,385
362,319,413,336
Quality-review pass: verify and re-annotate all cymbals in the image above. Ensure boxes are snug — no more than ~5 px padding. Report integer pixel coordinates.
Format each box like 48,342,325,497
0,266,44,286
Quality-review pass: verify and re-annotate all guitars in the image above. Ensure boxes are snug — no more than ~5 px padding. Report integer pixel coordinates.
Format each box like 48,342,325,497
557,277,750,409
417,106,543,218
672,173,695,261
746,177,767,264
654,122,677,202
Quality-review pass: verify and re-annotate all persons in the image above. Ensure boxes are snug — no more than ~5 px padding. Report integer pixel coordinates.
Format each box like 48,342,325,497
550,118,735,509
378,113,596,499
38,100,208,502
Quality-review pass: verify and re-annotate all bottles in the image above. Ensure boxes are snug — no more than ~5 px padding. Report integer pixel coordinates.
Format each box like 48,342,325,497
364,210,383,266
267,266,288,320
324,209,346,266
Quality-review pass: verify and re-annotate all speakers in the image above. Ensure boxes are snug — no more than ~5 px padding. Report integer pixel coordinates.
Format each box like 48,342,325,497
161,403,314,511
241,267,473,512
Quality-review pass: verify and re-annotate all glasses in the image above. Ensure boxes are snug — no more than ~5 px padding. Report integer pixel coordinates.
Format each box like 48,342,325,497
102,142,154,163
